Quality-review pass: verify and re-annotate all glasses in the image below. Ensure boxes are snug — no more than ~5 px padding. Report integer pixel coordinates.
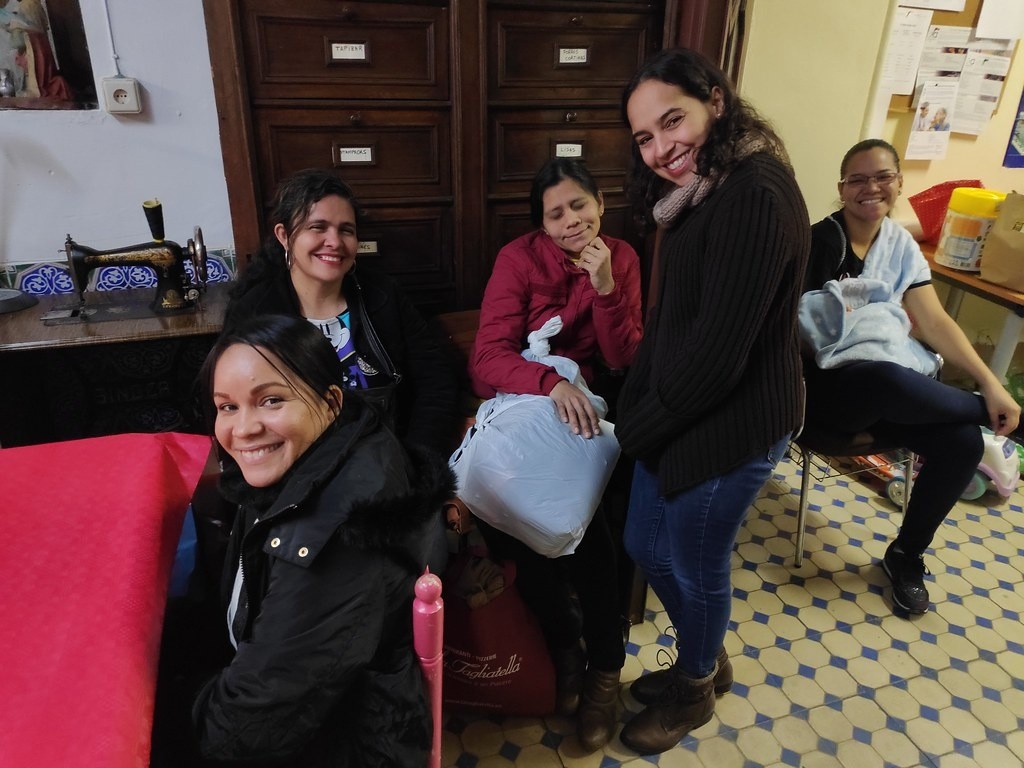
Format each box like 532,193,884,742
840,173,899,188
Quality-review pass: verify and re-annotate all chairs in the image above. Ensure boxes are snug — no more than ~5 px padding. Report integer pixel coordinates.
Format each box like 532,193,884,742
793,336,944,567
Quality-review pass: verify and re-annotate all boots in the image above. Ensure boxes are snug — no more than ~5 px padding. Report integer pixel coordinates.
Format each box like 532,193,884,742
630,626,733,704
549,634,589,717
620,649,718,754
577,664,621,752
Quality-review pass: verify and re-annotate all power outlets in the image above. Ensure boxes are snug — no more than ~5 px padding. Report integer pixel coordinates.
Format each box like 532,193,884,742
103,77,143,114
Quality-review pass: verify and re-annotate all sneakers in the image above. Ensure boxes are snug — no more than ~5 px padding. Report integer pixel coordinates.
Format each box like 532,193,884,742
976,394,1024,446
881,539,931,613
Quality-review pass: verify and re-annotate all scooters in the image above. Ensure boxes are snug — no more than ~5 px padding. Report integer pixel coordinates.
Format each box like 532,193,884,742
850,453,920,509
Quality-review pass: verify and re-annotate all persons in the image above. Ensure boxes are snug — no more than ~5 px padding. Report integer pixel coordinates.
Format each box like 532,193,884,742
619,48,810,755
221,168,458,462
805,139,1022,615
928,107,950,131
912,102,931,131
470,158,644,751
157,313,435,767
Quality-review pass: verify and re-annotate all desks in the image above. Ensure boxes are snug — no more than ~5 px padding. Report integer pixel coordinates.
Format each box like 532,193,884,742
0,281,231,352
0,428,214,768
916,240,1024,386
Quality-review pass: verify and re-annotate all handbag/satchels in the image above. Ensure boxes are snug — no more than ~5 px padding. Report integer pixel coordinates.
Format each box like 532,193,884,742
441,561,555,718
